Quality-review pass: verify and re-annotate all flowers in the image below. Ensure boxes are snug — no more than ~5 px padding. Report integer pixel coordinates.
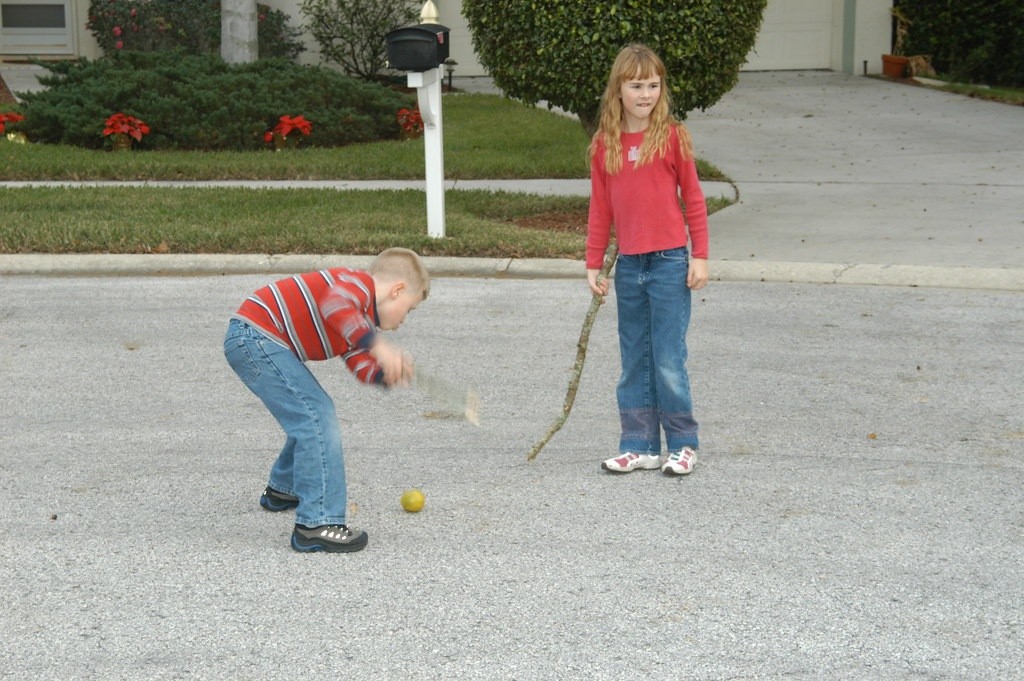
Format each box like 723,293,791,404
103,113,149,141
397,109,423,132
263,116,312,142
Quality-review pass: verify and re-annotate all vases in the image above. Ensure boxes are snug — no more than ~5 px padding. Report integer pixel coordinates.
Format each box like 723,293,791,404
400,125,420,141
109,133,135,151
273,134,303,149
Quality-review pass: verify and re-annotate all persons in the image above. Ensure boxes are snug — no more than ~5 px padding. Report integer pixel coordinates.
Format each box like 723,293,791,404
224,248,430,554
585,45,711,474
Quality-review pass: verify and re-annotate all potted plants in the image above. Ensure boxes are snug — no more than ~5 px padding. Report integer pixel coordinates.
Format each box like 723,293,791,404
881,7,914,78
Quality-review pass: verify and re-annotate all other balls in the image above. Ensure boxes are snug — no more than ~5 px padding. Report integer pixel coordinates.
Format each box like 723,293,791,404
401,489,426,513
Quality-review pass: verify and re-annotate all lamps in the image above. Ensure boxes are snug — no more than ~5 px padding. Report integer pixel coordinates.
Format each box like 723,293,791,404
445,58,458,90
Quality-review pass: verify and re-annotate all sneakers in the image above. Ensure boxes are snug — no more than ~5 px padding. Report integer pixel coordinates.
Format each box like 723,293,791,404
602,452,661,472
291,524,368,553
661,446,697,475
260,486,299,511
